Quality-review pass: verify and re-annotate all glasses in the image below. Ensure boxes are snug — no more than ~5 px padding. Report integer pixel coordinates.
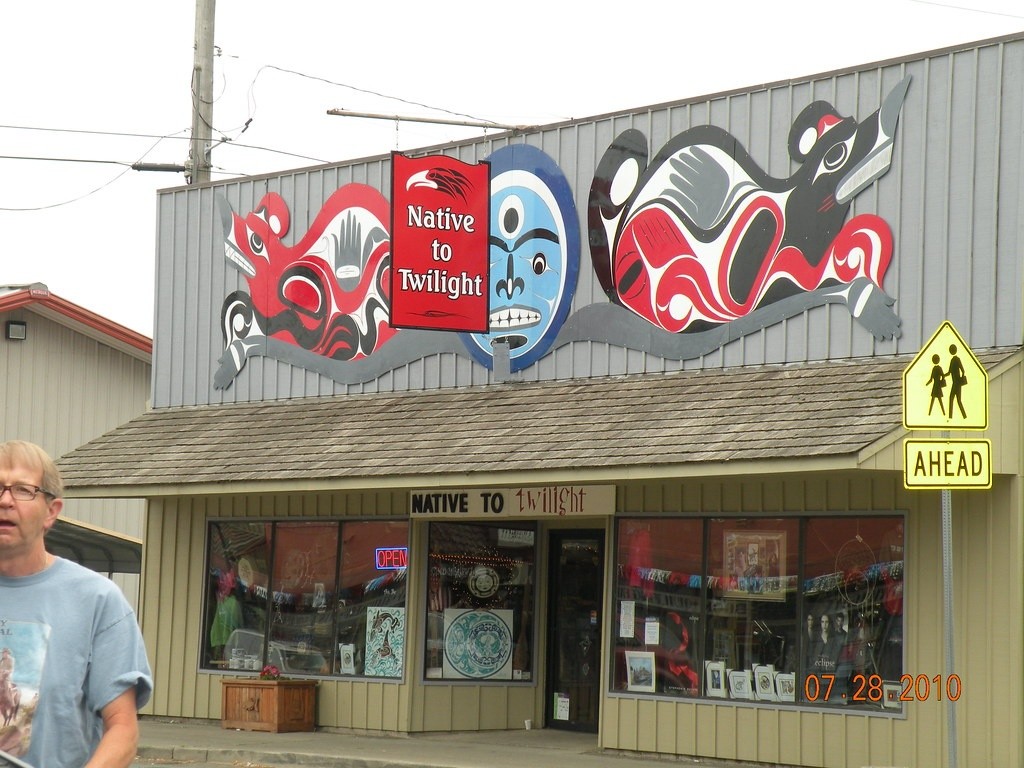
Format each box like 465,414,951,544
0,484,55,502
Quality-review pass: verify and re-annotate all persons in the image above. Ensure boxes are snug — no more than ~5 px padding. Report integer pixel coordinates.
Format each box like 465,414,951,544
0,441,155,768
210,595,244,661
727,538,778,594
714,670,720,689
785,681,793,693
808,612,847,672
760,675,770,689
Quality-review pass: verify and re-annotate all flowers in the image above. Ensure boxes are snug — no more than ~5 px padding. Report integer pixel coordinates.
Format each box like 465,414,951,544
260,664,286,680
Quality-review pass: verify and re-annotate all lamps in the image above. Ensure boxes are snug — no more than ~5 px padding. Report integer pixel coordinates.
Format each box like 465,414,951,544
6,320,27,340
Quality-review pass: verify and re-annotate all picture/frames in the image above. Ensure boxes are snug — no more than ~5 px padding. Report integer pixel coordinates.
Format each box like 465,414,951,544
722,529,787,602
339,643,355,674
704,661,795,703
623,649,657,694
880,680,902,711
